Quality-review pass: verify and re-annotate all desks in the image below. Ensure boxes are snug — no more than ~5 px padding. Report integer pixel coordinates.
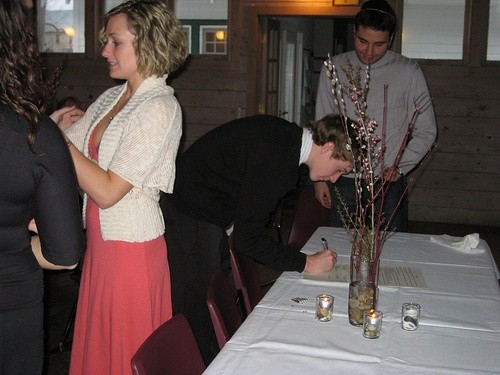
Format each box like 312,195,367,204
198,227,500,375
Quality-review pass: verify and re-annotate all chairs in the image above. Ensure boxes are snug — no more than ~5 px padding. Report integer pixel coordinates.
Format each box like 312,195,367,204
128,313,206,375
207,187,332,358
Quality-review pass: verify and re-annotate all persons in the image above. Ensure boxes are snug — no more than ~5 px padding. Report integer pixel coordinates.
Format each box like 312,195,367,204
159,114,368,370
314,0,437,233
28,0,188,375
0,0,82,375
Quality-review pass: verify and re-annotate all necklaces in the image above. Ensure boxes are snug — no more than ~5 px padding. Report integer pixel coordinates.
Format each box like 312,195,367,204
108,98,121,124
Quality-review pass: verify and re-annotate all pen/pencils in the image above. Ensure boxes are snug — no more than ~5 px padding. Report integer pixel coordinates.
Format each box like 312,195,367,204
321,237,328,250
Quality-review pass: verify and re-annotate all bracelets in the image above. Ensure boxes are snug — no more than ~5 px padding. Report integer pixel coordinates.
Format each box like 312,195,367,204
66,140,73,148
395,166,404,176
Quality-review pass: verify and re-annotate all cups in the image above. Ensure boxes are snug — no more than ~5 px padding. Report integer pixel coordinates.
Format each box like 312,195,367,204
316,295,334,322
362,310,383,339
402,303,421,331
